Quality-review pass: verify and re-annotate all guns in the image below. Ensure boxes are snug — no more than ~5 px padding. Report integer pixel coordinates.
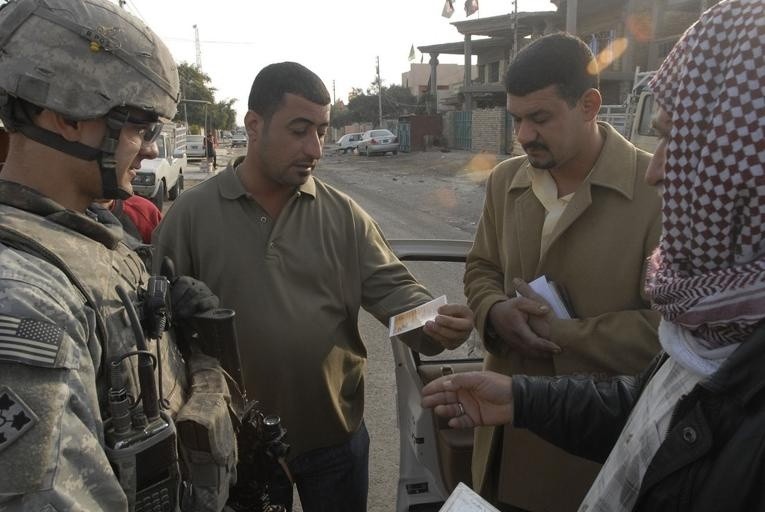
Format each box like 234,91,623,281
190,307,296,511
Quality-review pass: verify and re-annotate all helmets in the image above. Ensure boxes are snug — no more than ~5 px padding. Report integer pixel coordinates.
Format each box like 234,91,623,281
0,0,180,120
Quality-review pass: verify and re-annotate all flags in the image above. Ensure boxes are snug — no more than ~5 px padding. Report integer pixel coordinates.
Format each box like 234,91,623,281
464,0,480,17
441,0,455,18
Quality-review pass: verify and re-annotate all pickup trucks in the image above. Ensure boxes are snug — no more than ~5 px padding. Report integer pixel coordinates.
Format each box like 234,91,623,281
133,131,187,213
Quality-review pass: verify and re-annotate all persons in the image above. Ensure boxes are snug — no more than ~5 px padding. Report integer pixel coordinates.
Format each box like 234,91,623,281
0,0,261,512
206,135,217,168
203,130,211,157
462,30,665,512
419,0,765,512
150,61,476,512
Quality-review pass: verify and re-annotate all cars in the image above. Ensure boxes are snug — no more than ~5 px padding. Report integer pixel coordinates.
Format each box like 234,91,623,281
335,129,400,157
223,129,247,147
187,135,207,160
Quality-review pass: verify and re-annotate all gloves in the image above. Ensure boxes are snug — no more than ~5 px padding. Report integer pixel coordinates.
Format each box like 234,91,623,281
161,255,218,328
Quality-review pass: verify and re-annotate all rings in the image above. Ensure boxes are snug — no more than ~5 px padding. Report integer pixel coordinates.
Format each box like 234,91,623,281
459,403,465,416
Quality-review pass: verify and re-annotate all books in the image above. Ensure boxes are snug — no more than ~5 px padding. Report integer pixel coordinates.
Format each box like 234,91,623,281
515,275,575,319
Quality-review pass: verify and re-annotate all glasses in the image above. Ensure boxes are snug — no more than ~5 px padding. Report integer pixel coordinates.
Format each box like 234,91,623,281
125,117,165,146
